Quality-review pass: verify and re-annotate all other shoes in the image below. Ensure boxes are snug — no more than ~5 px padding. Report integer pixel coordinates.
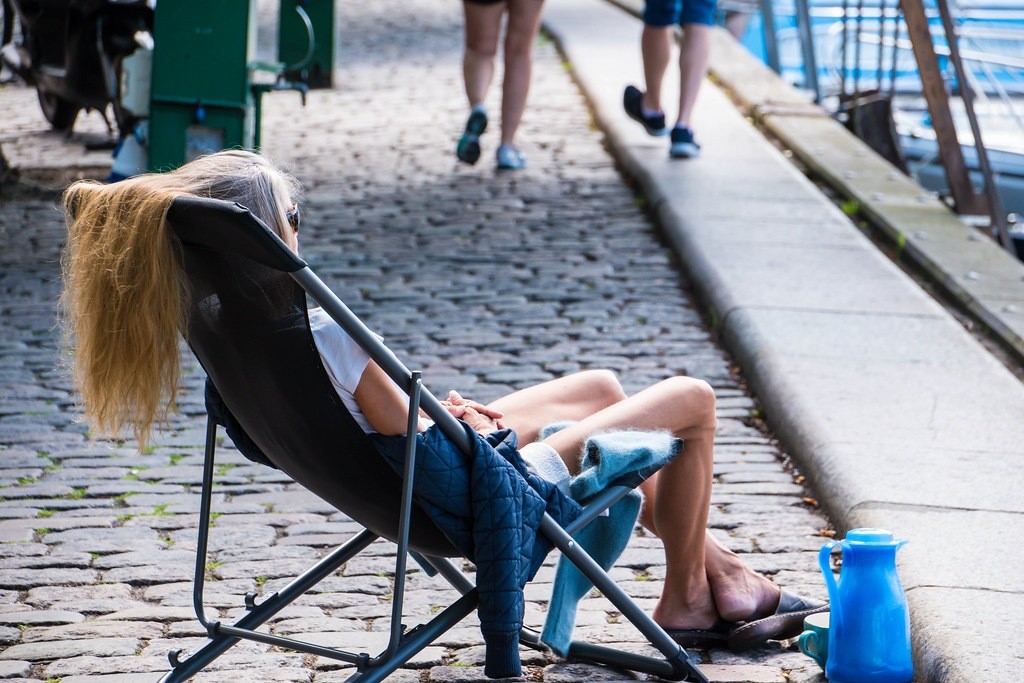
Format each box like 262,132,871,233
456,109,487,164
496,146,524,169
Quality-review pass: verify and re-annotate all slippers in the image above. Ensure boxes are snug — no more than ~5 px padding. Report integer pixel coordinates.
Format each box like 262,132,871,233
663,616,747,648
729,586,831,652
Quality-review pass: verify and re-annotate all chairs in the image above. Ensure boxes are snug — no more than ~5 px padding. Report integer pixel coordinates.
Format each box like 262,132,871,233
62,184,711,683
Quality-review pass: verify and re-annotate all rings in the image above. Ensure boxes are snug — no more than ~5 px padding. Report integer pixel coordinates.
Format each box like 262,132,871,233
463,403,470,407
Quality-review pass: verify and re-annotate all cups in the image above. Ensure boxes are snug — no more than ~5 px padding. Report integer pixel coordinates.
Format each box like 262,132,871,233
798,612,830,672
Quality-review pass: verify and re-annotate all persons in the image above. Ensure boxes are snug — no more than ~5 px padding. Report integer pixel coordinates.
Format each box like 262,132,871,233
457,0,543,170
624,0,718,158
58,149,830,651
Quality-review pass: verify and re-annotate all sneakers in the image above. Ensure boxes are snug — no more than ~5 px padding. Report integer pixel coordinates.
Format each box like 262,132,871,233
624,85,666,135
670,128,700,155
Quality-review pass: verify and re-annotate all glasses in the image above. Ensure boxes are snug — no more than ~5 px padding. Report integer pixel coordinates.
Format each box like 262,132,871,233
286,205,300,230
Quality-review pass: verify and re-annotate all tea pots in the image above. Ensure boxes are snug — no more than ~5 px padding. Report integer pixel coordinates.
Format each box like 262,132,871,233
818,528,914,683
